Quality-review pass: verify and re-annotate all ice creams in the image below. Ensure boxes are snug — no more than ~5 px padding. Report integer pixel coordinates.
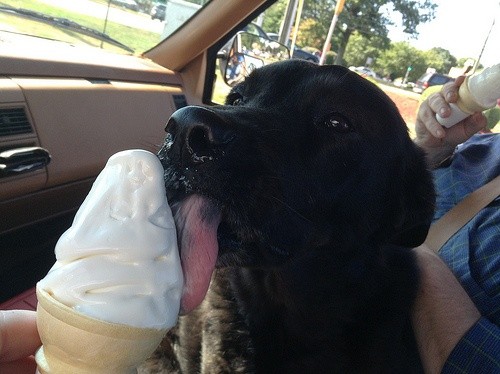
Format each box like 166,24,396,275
436,63,500,129
33,149,182,374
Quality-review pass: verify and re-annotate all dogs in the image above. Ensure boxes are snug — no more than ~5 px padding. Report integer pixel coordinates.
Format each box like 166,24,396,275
154,58,436,373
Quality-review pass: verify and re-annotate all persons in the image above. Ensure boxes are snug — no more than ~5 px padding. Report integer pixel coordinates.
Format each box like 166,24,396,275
0,310,42,374
401,72,500,373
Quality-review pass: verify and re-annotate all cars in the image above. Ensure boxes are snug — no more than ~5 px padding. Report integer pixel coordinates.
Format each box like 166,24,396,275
149,4,167,22
414,72,453,96
266,33,319,64
349,65,376,78
